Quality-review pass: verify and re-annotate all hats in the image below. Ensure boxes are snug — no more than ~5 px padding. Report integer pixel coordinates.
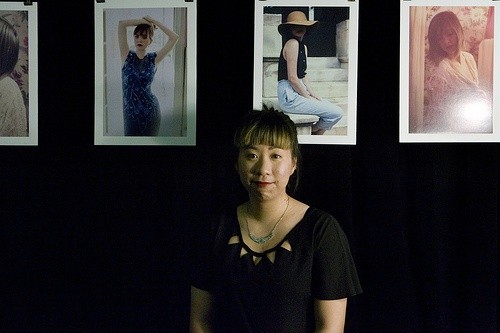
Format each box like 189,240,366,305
278,10,319,37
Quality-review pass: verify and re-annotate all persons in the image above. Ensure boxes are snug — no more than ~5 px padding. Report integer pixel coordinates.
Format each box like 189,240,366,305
426,11,479,131
117,16,179,136
0,17,27,136
276,11,343,135
191,110,362,333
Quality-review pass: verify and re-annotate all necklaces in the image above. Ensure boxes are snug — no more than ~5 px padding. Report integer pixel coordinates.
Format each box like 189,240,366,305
243,196,289,243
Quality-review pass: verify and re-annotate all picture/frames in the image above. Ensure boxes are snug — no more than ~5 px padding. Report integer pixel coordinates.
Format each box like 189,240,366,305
398,0,500,142
0,2,38,146
253,0,360,145
93,0,198,147
